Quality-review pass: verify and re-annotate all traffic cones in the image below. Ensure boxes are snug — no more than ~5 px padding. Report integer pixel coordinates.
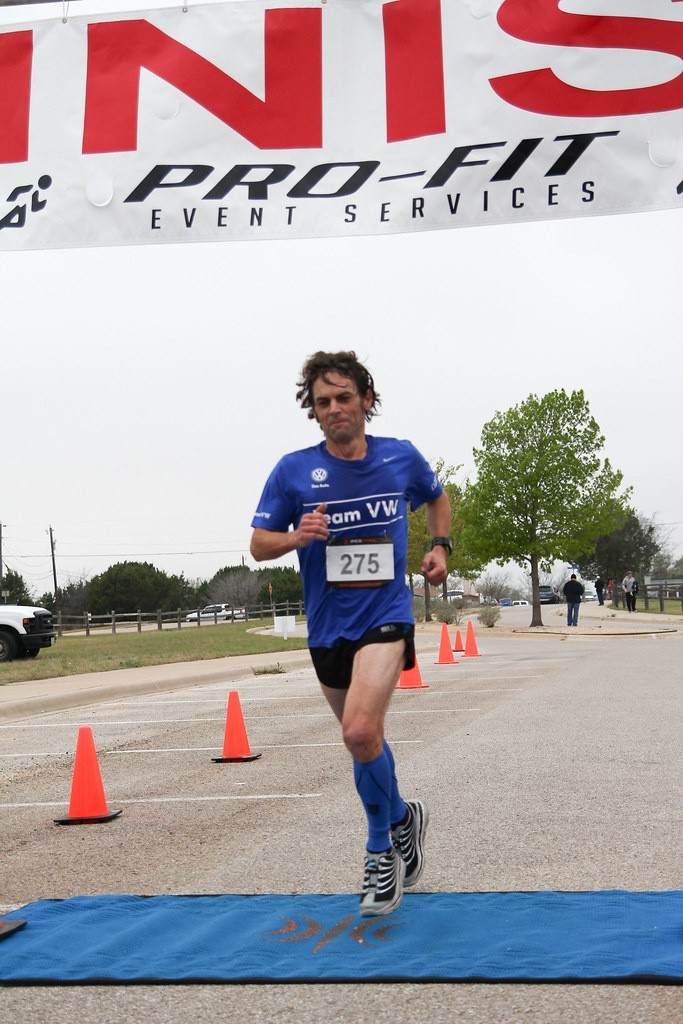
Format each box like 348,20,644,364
53,725,122,824
460,620,482,657
395,648,429,689
211,691,262,762
452,627,465,652
434,623,459,664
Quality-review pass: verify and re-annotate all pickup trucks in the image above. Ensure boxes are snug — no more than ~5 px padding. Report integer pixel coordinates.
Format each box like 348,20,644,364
186,604,249,622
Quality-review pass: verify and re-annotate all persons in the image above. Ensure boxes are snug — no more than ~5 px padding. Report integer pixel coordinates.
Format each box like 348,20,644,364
609,578,614,593
563,574,584,626
251,351,450,917
594,576,605,605
621,571,638,613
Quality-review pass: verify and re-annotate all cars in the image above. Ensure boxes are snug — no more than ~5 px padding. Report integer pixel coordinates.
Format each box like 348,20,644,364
430,590,529,606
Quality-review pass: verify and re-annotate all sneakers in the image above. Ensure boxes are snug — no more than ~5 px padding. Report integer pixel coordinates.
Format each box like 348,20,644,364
385,800,430,887
358,849,407,917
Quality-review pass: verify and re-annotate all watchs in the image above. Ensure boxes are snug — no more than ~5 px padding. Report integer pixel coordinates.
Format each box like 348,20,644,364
431,537,452,554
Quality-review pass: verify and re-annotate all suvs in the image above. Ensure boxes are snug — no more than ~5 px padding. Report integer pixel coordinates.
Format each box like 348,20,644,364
580,590,597,601
0,606,58,662
539,585,563,604
